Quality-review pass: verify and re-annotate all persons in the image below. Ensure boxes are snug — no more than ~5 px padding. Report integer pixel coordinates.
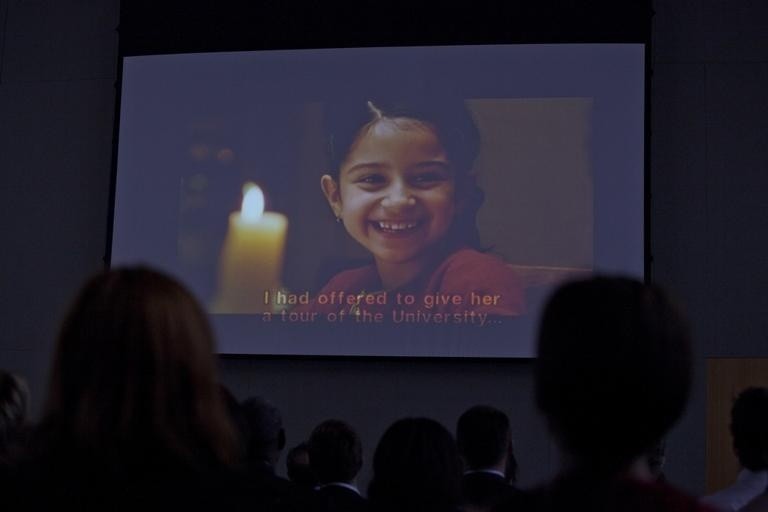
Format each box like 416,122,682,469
290,73,527,318
0,261,768,512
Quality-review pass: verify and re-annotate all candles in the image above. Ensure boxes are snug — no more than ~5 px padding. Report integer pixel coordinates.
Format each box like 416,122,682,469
216,183,287,314
